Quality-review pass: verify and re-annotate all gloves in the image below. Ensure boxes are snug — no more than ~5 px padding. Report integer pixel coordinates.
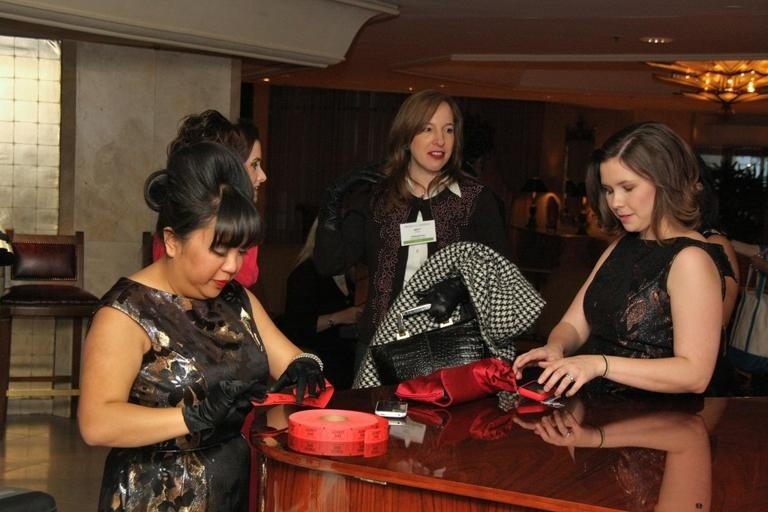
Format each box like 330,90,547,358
181,379,268,435
414,277,470,324
270,358,326,404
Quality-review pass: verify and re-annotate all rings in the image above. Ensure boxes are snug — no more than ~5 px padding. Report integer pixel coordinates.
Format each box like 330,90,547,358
565,374,574,383
561,431,571,437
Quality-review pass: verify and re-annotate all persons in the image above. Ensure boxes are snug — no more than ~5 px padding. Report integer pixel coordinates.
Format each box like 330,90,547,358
701,228,767,396
312,85,505,389
511,120,725,395
150,109,268,288
285,259,364,338
76,126,327,510
535,410,712,512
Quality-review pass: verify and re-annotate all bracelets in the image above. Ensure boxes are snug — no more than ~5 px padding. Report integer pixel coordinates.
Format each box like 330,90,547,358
596,424,603,448
293,352,323,373
601,354,608,377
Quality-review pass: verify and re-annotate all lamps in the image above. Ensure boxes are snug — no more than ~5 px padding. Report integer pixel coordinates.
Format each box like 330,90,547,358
519,176,548,228
649,62,768,117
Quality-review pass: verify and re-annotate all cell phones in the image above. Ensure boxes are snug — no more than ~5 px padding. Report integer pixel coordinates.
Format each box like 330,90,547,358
515,397,549,415
519,380,556,401
375,400,409,419
386,418,407,425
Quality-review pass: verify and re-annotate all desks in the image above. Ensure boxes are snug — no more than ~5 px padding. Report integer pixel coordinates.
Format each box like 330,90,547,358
244,376,766,512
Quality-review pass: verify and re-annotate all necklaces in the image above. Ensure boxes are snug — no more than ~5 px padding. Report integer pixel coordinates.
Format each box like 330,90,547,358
405,176,443,195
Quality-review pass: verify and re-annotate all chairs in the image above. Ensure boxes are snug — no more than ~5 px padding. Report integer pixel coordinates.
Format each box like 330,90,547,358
0,227,99,428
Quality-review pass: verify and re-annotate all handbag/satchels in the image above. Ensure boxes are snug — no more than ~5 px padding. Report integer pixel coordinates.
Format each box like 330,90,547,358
726,264,768,370
369,302,489,384
